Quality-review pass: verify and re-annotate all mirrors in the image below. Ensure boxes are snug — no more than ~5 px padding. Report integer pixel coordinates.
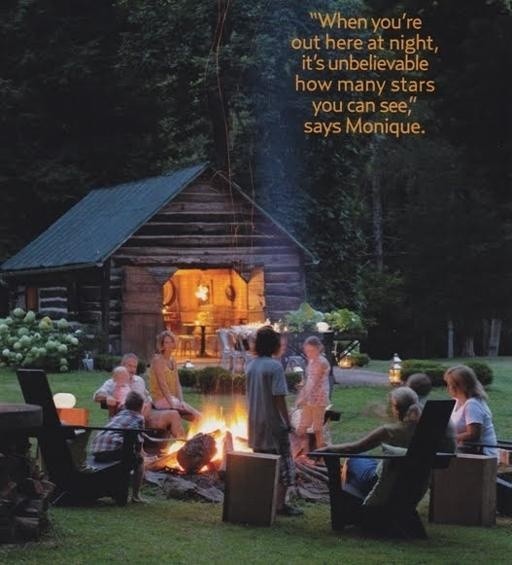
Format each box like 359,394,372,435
164,279,176,306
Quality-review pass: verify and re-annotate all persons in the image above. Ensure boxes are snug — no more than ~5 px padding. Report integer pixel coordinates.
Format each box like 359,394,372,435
149,330,201,422
270,334,289,372
94,353,186,439
112,367,131,411
309,387,422,493
407,373,457,454
444,366,500,467
294,336,332,463
247,326,304,516
91,391,150,504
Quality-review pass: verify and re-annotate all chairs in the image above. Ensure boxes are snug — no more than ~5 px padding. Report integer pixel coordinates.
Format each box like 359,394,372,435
16,369,166,506
457,440,512,516
216,329,250,374
304,404,342,452
306,400,456,538
101,366,194,457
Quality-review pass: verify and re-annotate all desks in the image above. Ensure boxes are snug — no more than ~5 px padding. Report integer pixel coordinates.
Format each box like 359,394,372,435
429,451,497,528
36,408,88,460
223,452,280,526
182,322,219,358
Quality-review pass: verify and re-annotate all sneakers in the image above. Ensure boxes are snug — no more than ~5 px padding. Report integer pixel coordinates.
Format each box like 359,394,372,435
277,504,305,516
130,496,150,504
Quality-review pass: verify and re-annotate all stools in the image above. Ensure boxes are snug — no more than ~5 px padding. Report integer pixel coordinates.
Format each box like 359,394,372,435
176,335,193,360
